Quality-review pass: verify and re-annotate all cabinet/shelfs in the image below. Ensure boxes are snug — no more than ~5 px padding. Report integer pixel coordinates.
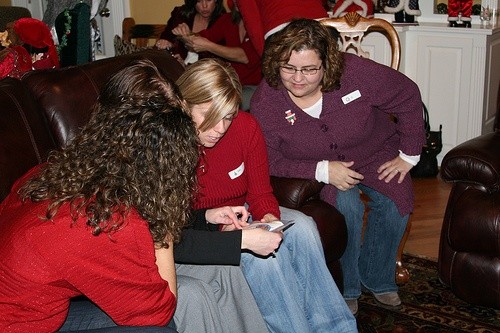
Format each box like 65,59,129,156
321,18,500,168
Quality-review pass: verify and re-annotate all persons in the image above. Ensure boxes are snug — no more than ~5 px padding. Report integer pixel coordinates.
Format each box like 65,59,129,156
93,58,269,333
151,0,228,65
174,57,359,333
171,0,264,89
248,18,426,318
1,91,207,333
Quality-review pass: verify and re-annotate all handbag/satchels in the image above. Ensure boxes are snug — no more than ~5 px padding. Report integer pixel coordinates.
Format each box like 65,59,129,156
409,100,443,179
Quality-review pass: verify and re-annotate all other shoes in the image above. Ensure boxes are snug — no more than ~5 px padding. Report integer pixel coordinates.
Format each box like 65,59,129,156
344,300,358,316
373,291,402,306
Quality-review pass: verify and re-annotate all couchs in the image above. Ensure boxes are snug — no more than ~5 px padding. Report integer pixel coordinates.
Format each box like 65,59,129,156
1,49,347,298
435,117,500,298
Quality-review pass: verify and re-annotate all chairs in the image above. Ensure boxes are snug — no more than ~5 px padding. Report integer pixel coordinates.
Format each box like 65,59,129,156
319,12,401,72
122,18,182,49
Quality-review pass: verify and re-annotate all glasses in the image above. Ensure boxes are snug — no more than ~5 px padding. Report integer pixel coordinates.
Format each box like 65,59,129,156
280,61,324,74
